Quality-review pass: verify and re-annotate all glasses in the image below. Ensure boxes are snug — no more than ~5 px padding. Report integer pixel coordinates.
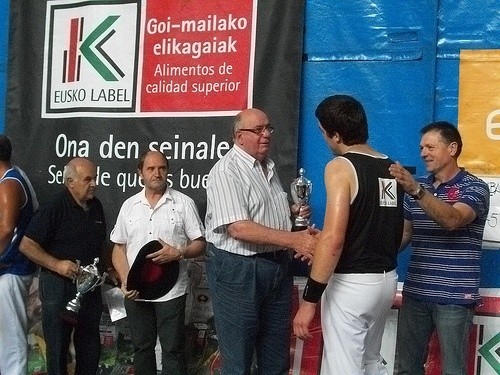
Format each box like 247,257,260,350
240,127,274,134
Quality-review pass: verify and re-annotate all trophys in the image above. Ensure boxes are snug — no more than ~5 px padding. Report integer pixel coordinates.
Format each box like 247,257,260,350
290,168,313,226
65,256,108,313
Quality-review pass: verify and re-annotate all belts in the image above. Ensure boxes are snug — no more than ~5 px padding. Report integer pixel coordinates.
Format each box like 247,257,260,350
257,250,288,259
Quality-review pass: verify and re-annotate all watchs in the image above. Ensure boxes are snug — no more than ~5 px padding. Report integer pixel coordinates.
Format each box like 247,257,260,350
411,185,425,200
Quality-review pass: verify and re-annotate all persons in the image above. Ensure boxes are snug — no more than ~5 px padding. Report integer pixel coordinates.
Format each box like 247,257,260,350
204,108,319,375
388,121,490,375
110,149,206,375
0,136,39,375
292,95,405,375
19,158,113,375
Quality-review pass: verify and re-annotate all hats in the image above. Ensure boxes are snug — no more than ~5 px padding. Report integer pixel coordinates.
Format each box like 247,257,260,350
126,240,178,300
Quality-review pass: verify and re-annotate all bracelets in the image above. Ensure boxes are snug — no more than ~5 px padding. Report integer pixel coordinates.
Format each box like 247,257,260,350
180,248,185,260
